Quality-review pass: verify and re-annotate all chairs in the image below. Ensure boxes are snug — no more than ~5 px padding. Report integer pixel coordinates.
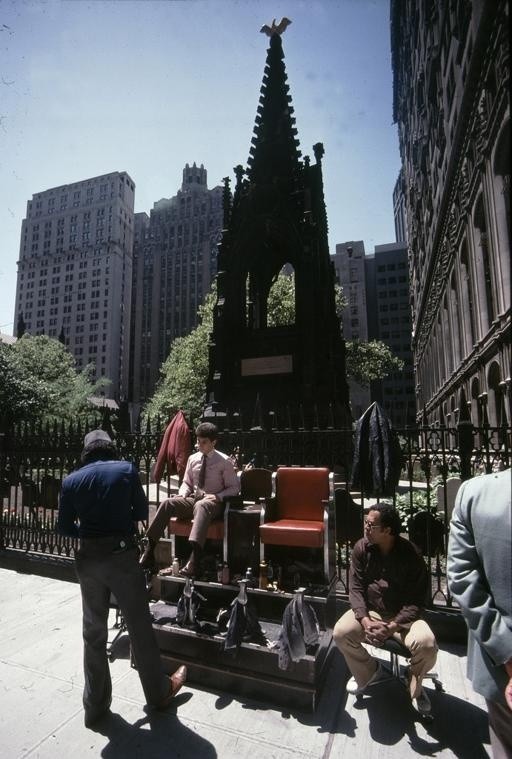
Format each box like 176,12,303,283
169,466,336,588
356,605,443,723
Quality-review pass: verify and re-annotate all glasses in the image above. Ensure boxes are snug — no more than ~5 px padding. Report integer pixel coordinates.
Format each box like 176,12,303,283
365,519,381,529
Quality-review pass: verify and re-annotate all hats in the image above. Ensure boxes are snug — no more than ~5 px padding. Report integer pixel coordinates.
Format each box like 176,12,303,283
84,429,113,448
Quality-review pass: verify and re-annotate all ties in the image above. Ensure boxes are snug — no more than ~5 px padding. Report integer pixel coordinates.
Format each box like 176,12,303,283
198,455,207,489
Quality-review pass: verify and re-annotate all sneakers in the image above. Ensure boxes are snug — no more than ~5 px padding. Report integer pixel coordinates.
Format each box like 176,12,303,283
412,691,432,713
346,661,381,693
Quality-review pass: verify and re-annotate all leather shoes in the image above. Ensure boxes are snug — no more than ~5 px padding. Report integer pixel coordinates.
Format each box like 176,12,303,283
170,665,187,696
178,561,194,575
139,554,155,567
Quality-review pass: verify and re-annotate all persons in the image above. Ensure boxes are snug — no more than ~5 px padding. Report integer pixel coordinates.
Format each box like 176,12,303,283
332,502,439,715
56,428,189,728
137,421,241,576
445,467,512,759
